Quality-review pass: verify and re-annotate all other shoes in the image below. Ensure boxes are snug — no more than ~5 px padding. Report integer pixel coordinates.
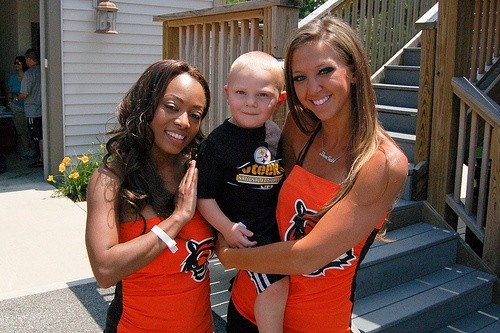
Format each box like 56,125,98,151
18,154,33,160
27,160,42,168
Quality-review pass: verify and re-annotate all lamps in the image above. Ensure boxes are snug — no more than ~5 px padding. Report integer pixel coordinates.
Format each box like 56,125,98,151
95,0,119,34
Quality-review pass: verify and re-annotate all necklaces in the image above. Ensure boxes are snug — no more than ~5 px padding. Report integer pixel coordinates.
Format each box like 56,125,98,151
319,130,346,163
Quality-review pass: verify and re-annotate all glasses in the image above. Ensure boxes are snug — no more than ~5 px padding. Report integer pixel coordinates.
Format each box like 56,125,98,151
14,62,22,65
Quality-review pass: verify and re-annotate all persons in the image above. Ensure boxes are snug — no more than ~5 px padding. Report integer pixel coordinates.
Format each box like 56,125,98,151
86,60,216,333
196,50,291,333
215,16,408,333
7,49,44,168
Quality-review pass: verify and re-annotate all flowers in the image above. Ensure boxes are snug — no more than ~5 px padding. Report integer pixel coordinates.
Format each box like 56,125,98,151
46,143,113,203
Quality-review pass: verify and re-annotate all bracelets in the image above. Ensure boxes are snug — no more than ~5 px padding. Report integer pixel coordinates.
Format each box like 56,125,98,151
15,96,19,102
151,225,178,254
